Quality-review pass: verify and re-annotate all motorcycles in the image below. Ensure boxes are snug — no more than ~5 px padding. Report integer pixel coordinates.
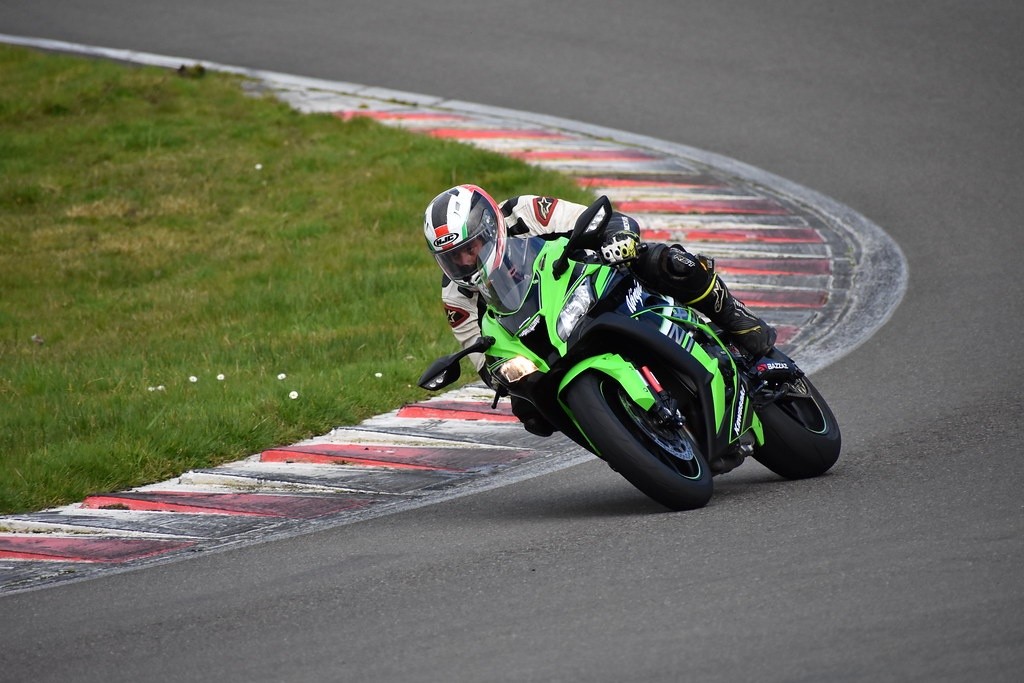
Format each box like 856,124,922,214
414,194,843,512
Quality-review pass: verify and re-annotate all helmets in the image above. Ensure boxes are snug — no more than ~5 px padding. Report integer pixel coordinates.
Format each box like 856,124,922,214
424,187,507,292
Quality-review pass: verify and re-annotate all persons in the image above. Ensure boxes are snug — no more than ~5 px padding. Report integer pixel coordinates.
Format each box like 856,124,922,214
423,184,777,388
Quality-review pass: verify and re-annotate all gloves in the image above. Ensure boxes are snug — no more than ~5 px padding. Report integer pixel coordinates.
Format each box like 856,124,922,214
600,235,637,265
490,377,509,397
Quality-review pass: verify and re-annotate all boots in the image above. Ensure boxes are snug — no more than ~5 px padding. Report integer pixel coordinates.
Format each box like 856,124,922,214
685,271,775,355
